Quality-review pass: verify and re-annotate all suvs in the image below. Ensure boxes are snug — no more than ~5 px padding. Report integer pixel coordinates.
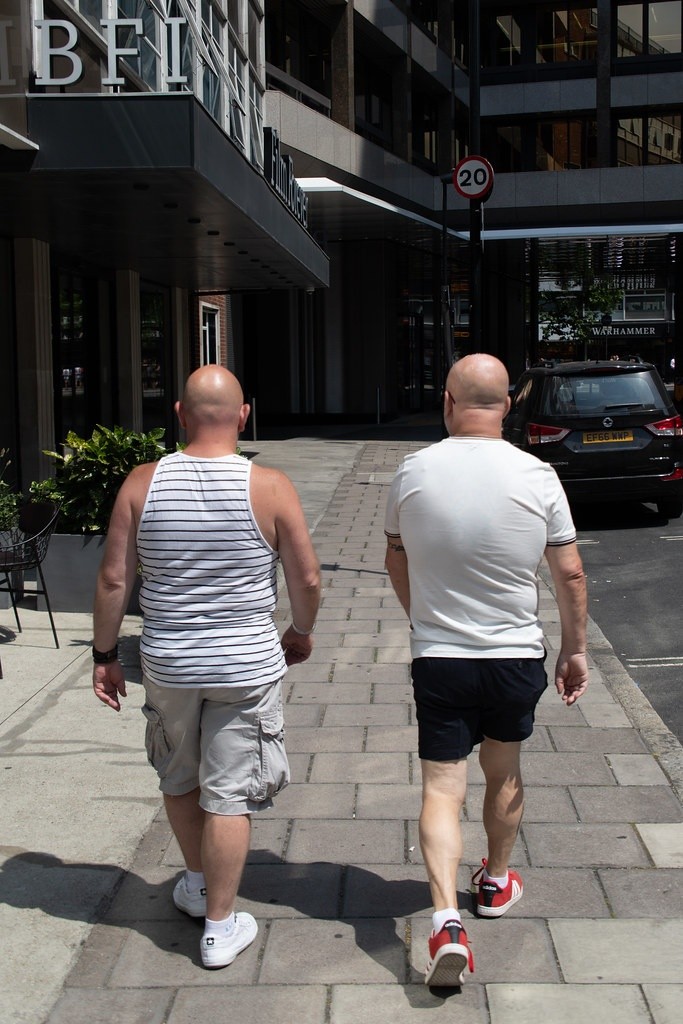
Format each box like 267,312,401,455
502,356,683,519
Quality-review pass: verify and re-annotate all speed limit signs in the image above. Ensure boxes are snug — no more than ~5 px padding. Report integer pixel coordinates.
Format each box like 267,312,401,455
453,154,495,199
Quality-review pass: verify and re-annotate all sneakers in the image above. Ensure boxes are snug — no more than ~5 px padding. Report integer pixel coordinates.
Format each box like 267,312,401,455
424,919,474,987
200,911,258,967
173,876,207,917
471,857,523,916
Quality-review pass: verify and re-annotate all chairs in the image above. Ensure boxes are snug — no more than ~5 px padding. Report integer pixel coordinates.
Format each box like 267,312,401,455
0,497,58,679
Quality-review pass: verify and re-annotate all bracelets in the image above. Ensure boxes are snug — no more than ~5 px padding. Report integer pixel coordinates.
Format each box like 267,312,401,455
292,618,316,635
92,642,119,664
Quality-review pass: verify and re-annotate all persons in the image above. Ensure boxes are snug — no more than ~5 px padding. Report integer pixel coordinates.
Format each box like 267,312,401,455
92,364,322,969
386,353,589,986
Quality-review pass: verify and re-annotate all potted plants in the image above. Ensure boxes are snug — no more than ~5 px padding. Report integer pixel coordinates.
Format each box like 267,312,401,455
0,422,258,614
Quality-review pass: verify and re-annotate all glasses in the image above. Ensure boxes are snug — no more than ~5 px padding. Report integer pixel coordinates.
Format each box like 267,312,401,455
440,389,455,404
